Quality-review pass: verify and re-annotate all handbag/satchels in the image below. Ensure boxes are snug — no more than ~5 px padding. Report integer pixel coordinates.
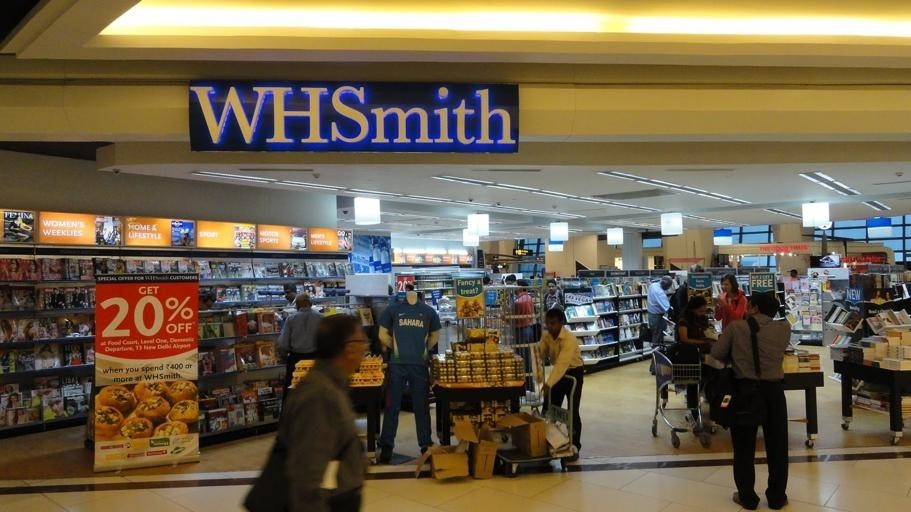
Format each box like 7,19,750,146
533,323,541,342
705,367,748,423
242,434,340,512
638,323,651,341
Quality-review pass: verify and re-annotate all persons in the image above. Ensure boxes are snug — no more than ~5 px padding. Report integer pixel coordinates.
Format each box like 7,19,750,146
377,290,442,466
388,283,415,303
8,216,28,241
645,268,798,510
96,226,120,246
483,274,565,395
535,308,586,463
240,310,369,511
181,233,191,246
343,231,351,251
273,294,326,435
235,231,256,249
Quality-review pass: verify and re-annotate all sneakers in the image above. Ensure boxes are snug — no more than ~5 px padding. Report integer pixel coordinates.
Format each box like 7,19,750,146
768,494,787,510
733,491,758,509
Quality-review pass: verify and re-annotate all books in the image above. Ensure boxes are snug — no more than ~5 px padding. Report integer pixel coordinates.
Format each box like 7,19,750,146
200,256,350,432
2,259,95,426
784,259,910,419
98,257,199,273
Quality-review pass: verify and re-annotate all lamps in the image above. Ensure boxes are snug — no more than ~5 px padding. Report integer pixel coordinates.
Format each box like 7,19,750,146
548,240,563,251
606,226,624,245
660,213,683,236
353,196,380,227
713,228,732,245
463,228,479,247
802,201,829,228
867,216,892,238
467,210,489,236
549,221,569,242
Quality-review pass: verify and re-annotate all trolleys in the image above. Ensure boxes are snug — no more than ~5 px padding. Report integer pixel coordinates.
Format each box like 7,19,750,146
648,343,711,453
495,370,582,474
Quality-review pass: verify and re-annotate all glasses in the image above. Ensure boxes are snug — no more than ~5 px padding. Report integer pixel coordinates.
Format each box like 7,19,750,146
347,338,372,345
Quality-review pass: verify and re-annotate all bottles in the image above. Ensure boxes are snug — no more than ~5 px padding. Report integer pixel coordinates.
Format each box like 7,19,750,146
368,244,391,273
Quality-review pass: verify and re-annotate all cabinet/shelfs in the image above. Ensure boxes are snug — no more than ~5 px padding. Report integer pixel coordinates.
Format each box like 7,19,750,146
776,271,911,348
393,273,652,365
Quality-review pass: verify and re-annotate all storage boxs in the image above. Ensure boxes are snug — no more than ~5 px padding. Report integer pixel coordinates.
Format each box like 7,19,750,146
414,446,470,480
496,412,547,457
453,421,498,479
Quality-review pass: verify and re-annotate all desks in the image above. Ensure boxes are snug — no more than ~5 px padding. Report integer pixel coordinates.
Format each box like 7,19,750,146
703,371,824,448
287,364,390,453
432,386,525,444
834,361,911,444
